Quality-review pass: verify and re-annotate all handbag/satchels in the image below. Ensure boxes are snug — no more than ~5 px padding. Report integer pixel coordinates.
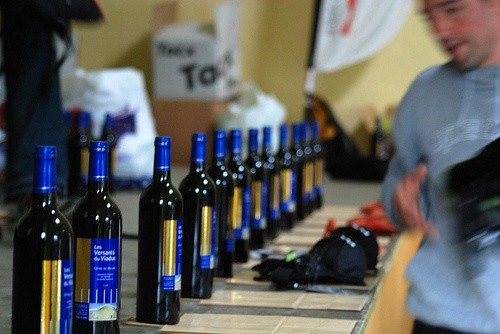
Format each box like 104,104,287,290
73,68,163,191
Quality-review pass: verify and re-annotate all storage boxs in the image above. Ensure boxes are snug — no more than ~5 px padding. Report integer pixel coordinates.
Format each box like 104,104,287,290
151,3,245,98
151,98,236,163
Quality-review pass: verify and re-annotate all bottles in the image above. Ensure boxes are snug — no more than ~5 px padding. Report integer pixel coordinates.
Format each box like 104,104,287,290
371,116,386,161
69,139,123,334
64,110,113,197
178,120,325,299
135,136,185,325
11,145,75,334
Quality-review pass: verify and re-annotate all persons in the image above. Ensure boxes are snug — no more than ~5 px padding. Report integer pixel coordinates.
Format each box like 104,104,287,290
381,0,500,334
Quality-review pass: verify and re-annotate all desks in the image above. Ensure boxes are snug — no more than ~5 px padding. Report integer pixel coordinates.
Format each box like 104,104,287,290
0,167,422,334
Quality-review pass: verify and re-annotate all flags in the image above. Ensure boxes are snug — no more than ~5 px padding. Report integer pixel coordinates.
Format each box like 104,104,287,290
313,0,413,75
